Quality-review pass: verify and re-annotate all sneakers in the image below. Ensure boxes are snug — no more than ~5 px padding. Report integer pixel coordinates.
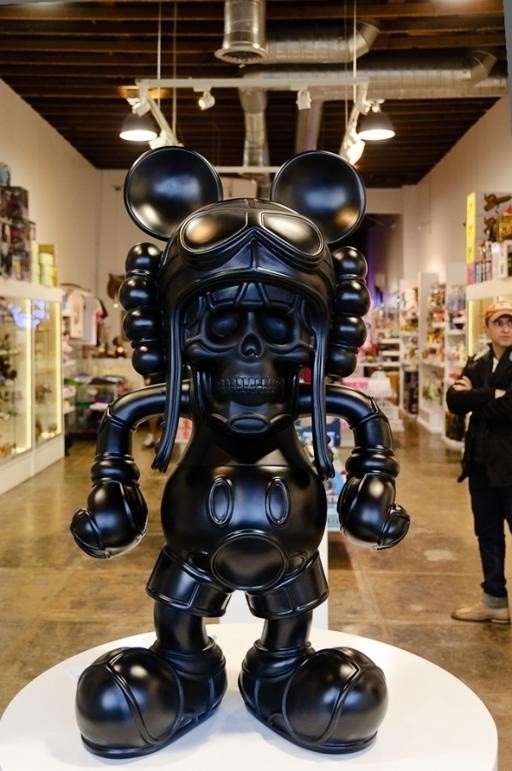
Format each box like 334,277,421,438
450,599,512,624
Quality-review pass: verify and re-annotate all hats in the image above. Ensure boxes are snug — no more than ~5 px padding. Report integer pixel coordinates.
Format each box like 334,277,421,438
484,300,512,323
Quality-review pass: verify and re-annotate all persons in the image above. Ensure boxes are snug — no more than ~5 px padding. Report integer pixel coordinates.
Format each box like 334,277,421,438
441,301,511,630
142,371,169,453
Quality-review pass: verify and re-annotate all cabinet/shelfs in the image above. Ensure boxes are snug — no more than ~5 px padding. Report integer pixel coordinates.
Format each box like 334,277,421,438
363,270,510,448
1,278,65,495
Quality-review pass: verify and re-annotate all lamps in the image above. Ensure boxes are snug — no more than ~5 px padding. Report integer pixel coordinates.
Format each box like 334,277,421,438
117,75,396,142
